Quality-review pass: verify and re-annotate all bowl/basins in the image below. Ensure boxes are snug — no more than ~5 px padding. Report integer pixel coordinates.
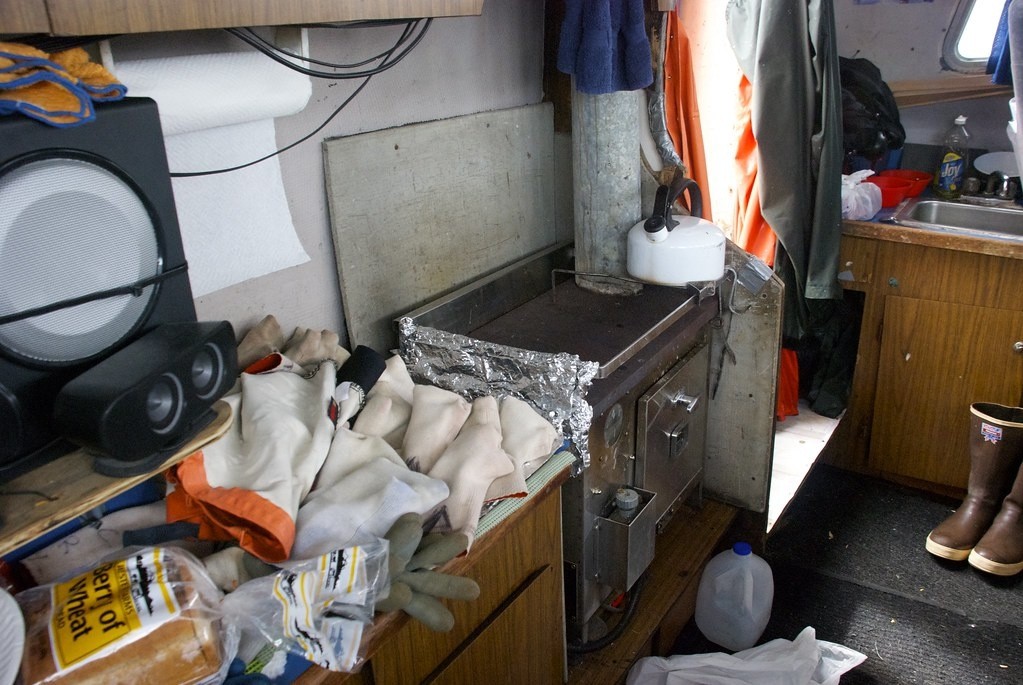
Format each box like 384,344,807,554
879,170,933,198
862,174,914,208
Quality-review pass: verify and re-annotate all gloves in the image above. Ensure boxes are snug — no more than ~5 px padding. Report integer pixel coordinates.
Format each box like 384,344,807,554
226,513,479,633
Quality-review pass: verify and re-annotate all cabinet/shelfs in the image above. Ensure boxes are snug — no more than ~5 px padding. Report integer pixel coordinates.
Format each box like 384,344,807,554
839,221,1023,506
288,449,580,685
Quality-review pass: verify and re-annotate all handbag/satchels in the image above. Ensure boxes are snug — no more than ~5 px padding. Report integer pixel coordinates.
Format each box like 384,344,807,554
839,168,882,221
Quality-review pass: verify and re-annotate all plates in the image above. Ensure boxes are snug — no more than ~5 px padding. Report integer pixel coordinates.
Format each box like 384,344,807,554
973,151,1019,177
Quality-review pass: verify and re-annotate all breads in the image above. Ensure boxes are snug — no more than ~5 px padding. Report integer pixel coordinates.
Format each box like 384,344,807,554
12,546,223,685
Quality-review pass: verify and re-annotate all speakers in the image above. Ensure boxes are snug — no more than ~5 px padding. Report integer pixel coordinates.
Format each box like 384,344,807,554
53,317,239,479
0,94,202,485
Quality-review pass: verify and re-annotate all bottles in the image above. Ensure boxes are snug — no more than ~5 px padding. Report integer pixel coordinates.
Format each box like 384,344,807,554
608,488,647,525
695,542,774,652
934,115,971,200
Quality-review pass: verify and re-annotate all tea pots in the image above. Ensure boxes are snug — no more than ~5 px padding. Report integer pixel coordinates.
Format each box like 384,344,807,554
626,177,727,287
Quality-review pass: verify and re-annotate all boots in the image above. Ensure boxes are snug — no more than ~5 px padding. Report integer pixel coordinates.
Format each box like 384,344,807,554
924,401,1023,562
968,461,1023,577
